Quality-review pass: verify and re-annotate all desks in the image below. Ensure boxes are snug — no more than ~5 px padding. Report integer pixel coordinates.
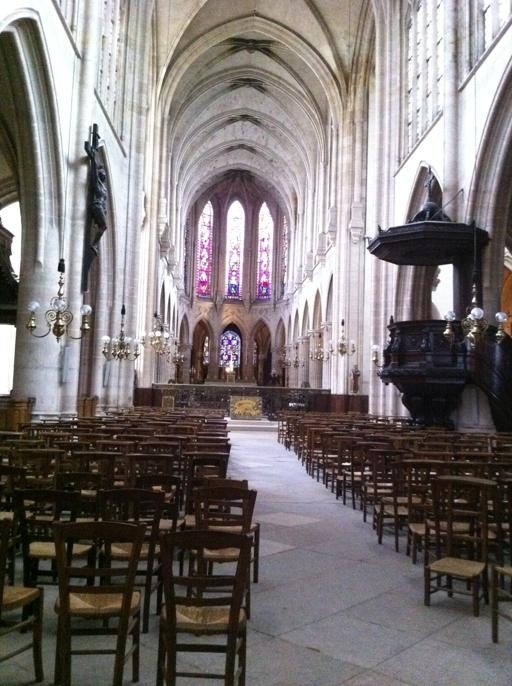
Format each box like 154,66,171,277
309,394,369,414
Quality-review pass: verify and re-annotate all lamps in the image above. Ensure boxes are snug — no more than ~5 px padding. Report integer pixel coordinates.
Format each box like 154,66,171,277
26,1,93,346
443,0,509,352
145,1,188,365
101,1,140,361
